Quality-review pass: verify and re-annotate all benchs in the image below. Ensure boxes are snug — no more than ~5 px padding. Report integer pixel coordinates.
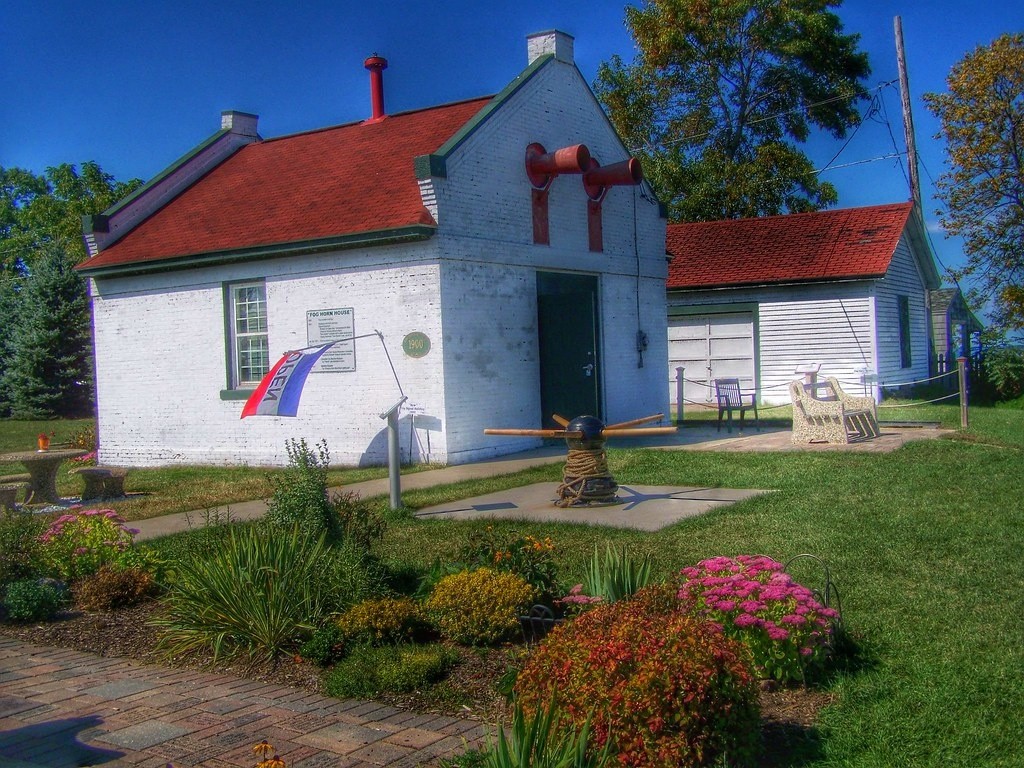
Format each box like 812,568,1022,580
67,466,129,501
0,473,34,509
790,377,880,444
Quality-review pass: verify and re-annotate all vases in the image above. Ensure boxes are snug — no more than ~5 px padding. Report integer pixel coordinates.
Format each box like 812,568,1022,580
38,439,51,453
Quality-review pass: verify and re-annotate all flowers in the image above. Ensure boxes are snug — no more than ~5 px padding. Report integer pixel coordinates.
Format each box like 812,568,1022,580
39,430,55,439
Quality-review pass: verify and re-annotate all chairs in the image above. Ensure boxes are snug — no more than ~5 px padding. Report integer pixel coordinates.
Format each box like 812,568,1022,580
714,377,760,434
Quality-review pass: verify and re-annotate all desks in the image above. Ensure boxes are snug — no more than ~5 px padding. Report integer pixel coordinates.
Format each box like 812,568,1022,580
0,449,90,509
